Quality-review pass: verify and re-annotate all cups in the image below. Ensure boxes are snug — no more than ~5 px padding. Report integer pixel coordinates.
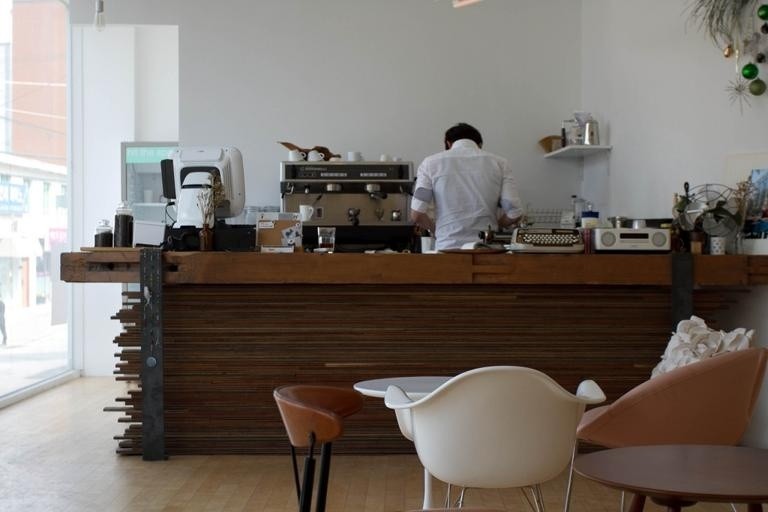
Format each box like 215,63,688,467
287,149,325,163
299,205,313,221
710,237,726,256
347,151,366,163
316,227,337,253
420,237,434,254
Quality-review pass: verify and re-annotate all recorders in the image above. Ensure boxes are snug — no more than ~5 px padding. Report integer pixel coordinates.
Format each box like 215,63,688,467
591,227,671,253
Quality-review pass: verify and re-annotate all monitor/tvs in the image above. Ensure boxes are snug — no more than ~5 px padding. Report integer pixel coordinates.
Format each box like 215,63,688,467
172,145,245,252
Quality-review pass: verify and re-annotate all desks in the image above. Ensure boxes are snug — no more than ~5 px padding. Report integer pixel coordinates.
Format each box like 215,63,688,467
574,446,768,512
353,376,452,401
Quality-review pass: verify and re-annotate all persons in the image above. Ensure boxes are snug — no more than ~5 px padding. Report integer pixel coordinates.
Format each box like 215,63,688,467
410,122,526,251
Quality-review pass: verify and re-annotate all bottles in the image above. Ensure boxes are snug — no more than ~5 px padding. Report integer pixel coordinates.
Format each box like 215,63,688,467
583,118,600,146
243,205,260,224
95,202,133,248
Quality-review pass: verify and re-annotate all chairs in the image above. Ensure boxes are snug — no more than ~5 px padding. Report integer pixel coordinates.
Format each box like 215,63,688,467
565,347,768,512
272,383,366,512
383,365,606,512
